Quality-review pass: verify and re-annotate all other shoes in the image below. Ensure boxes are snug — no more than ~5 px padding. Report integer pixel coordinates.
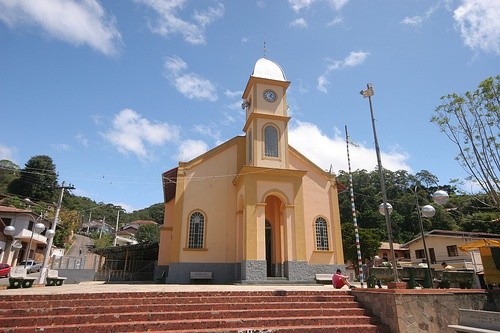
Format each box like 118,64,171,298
349,284,357,289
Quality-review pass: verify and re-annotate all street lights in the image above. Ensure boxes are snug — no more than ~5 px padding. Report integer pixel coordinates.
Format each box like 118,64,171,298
359,83,403,282
3,213,55,269
378,176,450,288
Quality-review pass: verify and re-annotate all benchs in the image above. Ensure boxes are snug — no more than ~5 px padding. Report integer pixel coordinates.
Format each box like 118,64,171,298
315,273,334,285
8,267,38,289
154,270,166,284
363,267,405,286
190,272,213,285
46,269,67,286
399,267,436,287
433,271,477,289
447,308,500,333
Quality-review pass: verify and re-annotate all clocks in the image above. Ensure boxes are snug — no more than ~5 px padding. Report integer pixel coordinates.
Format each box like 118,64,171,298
263,88,279,103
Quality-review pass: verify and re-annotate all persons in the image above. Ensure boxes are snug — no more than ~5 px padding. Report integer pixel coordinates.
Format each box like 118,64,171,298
441,262,456,289
332,268,357,289
365,256,388,288
418,259,428,268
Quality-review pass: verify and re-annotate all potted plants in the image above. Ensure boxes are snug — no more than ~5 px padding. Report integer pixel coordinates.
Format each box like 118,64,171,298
386,278,407,289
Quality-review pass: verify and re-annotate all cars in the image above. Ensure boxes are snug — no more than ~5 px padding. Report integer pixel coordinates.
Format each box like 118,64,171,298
0,262,11,278
20,259,42,274
